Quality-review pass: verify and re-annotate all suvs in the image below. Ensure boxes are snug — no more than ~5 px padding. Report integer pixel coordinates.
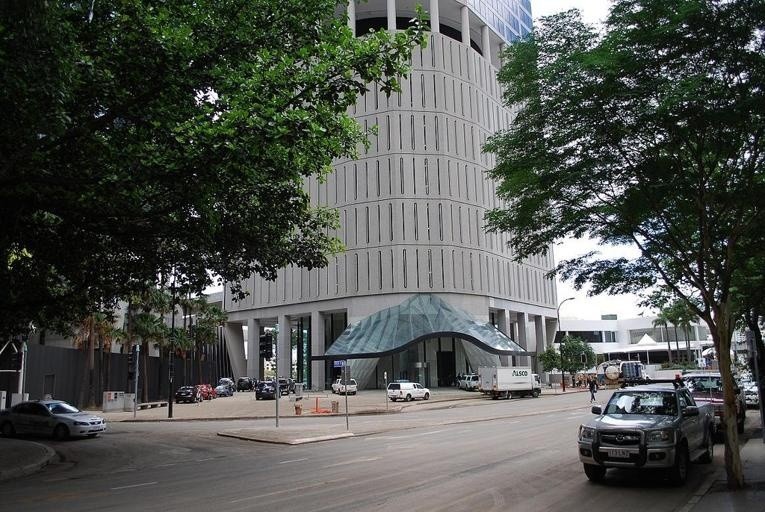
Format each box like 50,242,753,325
330,378,358,395
386,378,432,402
677,367,758,439
457,373,480,391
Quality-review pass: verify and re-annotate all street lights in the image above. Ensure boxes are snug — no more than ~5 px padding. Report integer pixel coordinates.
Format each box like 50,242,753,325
557,296,575,392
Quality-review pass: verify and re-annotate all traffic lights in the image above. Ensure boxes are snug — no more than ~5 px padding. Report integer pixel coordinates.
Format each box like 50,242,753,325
126,350,135,381
258,325,274,362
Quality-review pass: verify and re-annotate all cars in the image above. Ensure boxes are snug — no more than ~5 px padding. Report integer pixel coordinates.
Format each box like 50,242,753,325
0,399,109,442
174,374,297,404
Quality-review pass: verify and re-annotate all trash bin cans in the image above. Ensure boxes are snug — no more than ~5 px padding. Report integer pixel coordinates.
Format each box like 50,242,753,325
124,393,136,412
294,383,304,396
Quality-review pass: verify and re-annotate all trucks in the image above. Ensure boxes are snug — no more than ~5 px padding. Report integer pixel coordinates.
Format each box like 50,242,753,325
477,364,542,401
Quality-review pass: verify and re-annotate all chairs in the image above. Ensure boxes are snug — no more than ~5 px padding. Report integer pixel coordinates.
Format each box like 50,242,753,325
615,395,676,414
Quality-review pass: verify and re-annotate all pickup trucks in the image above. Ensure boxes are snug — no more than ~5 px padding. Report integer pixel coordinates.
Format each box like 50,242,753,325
573,373,718,483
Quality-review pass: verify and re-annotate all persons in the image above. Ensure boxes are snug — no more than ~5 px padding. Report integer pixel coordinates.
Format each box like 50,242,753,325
587,375,598,403
